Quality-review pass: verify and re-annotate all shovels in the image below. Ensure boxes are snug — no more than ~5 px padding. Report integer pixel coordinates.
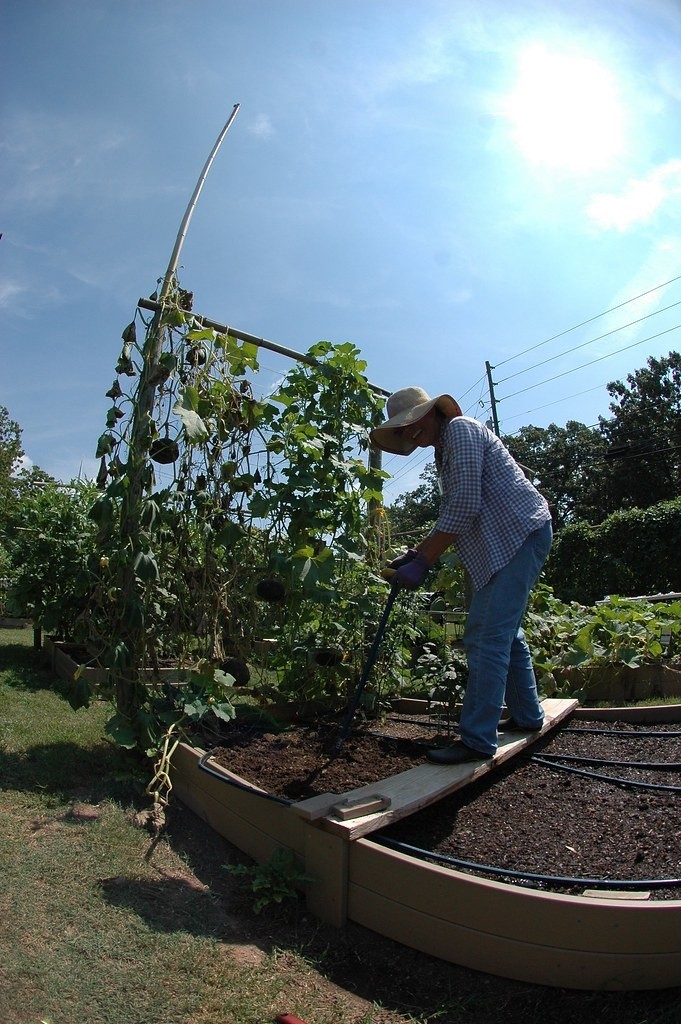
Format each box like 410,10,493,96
279,571,406,801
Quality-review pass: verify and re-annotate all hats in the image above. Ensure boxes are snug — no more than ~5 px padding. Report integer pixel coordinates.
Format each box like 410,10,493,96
369,388,463,456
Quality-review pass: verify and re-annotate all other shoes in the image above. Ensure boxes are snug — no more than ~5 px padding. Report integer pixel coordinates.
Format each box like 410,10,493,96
497,715,542,732
425,740,492,766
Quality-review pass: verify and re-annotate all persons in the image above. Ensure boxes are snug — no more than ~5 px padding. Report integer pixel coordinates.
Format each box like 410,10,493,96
368,385,554,766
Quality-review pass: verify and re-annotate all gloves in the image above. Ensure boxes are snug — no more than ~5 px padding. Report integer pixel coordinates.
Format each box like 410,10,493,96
382,549,418,586
391,553,432,589
